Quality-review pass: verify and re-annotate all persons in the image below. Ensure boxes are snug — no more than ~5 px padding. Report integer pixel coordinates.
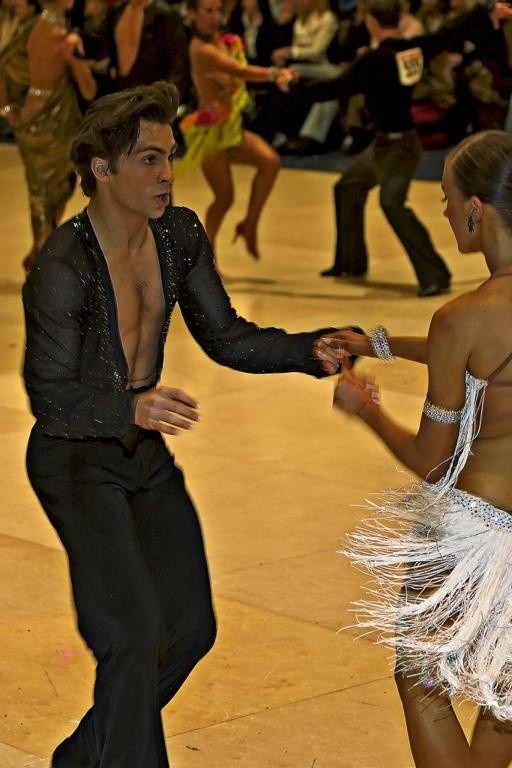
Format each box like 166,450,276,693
308,127,512,766
1,1,511,305
15,78,368,767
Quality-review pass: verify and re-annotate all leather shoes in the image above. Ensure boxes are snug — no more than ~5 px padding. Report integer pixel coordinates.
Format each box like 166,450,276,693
272,124,372,155
320,261,369,278
417,274,450,297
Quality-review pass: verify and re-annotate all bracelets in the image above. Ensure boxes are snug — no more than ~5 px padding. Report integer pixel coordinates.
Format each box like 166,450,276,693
369,323,397,365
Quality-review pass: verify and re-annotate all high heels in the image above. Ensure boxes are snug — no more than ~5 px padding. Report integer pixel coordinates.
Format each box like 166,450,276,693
231,220,261,261
